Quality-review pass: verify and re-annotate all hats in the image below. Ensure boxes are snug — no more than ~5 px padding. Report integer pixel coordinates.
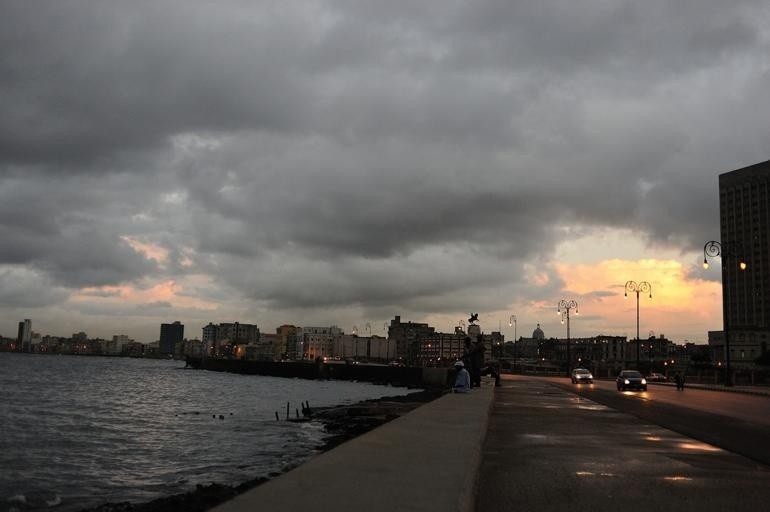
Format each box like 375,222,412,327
454,362,465,367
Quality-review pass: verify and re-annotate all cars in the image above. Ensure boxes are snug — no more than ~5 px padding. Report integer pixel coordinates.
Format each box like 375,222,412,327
320,356,369,366
646,373,667,382
388,361,400,367
616,370,647,392
571,368,594,384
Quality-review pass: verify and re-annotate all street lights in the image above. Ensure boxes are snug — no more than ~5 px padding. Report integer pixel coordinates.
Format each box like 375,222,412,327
352,325,359,336
624,281,653,371
384,321,389,331
639,339,653,371
557,300,579,377
508,315,516,377
703,240,747,387
365,322,372,336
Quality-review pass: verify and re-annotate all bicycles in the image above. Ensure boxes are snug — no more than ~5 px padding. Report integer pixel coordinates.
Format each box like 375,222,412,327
673,379,684,392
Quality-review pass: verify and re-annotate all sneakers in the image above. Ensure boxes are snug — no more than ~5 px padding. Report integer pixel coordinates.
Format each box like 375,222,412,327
472,384,480,387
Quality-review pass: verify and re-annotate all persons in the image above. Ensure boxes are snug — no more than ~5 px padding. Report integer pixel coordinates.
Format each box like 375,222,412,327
448,341,502,393
675,369,685,391
